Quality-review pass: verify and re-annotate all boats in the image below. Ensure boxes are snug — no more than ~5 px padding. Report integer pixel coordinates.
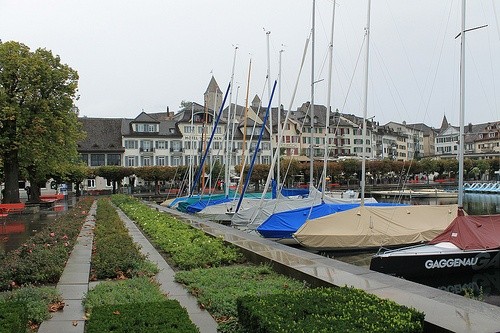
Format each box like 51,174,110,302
159,43,248,213
230,2,382,228
196,27,311,221
290,2,466,252
253,1,416,246
366,0,500,283
